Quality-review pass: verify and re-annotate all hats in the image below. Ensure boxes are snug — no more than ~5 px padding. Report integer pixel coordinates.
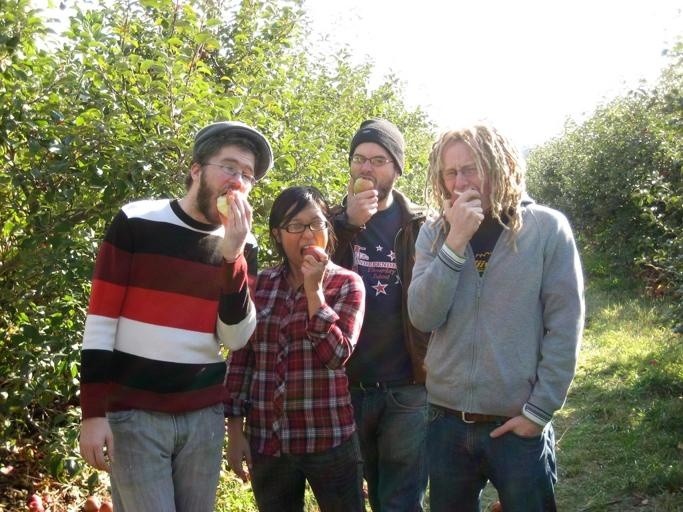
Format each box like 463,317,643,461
193,121,273,181
352,118,405,176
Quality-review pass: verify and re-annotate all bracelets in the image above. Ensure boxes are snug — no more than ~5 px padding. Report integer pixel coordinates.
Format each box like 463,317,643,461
225,251,241,260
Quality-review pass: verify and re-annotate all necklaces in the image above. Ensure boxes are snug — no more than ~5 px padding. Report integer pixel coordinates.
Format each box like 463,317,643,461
288,265,301,287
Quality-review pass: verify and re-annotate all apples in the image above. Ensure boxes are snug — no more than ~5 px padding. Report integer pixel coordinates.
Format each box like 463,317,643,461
301,246,324,262
353,177,373,195
451,190,462,206
216,193,232,218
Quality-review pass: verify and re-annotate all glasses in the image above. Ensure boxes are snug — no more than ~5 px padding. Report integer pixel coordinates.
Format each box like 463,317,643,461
199,159,257,188
348,155,392,167
279,222,326,234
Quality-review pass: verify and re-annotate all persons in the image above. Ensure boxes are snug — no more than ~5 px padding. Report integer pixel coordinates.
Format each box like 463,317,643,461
218,185,366,510
76,119,270,511
319,119,447,511
408,120,586,511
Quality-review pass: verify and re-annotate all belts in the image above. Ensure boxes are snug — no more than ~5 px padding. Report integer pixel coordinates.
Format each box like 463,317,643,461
348,376,412,389
437,405,503,424
